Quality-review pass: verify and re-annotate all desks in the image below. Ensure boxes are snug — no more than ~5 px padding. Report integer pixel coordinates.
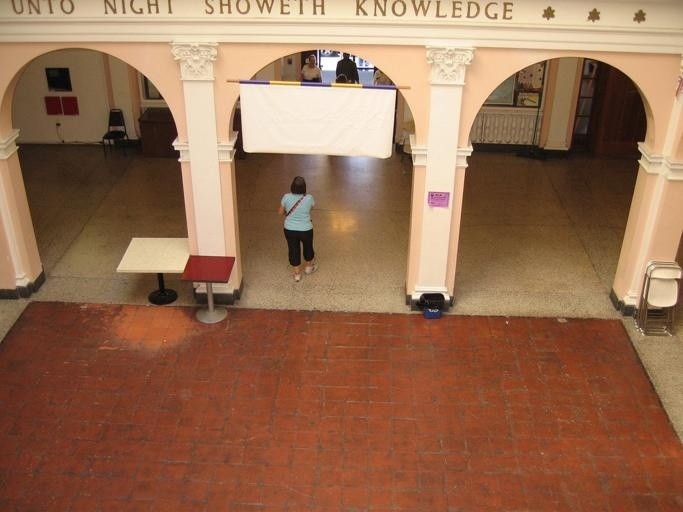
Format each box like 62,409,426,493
114,235,237,325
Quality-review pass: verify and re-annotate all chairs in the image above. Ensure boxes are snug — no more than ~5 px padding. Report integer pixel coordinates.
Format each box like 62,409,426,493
100,108,132,157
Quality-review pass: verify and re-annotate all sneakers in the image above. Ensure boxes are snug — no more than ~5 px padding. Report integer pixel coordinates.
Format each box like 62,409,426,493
304,262,318,274
294,272,302,282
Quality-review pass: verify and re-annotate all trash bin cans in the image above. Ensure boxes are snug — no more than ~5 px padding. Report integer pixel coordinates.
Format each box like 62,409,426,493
422,293,445,319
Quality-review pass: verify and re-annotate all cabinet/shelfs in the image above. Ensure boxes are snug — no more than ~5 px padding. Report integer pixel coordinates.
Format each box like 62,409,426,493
138,106,247,159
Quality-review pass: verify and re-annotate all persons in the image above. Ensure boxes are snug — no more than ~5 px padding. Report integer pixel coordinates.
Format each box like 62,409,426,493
373,69,395,86
299,54,321,83
279,176,314,282
336,53,368,84
